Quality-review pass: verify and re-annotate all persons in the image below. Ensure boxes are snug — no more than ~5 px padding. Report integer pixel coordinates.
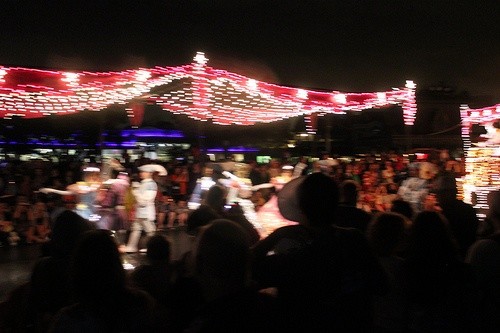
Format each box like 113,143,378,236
362,210,417,332
1,148,88,332
50,229,159,332
89,142,500,271
248,173,390,330
448,242,499,332
132,237,184,303
388,212,458,332
149,219,282,333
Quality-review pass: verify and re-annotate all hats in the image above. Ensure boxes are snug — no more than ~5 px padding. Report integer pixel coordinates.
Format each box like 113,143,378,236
137,163,168,177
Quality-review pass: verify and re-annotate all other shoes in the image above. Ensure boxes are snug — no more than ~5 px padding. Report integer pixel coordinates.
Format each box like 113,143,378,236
118,245,147,254
